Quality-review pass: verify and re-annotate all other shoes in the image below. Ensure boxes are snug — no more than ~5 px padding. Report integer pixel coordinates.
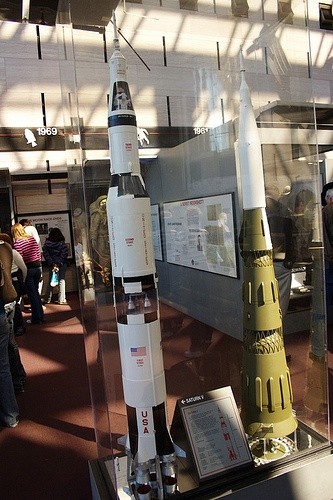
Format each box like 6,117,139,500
59,302,69,305
13,383,24,393
9,416,19,428
26,320,31,323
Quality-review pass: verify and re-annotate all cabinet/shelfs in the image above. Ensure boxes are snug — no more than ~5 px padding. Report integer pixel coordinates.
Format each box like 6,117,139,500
42,264,77,295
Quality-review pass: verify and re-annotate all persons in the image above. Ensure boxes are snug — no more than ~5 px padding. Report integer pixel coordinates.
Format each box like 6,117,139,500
325,190,333,292
42,228,69,304
0,246,20,396
197,234,203,251
0,218,47,335
292,189,316,258
239,185,295,362
0,240,27,428
216,212,232,272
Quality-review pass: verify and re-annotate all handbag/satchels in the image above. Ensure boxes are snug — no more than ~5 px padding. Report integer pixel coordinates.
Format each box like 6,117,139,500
50,271,60,287
